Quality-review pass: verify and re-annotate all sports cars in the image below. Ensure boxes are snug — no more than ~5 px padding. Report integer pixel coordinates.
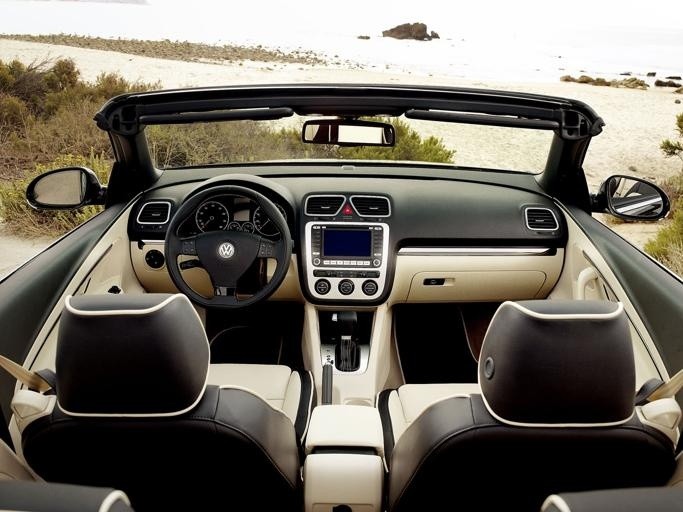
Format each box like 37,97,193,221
0,82,681,512
